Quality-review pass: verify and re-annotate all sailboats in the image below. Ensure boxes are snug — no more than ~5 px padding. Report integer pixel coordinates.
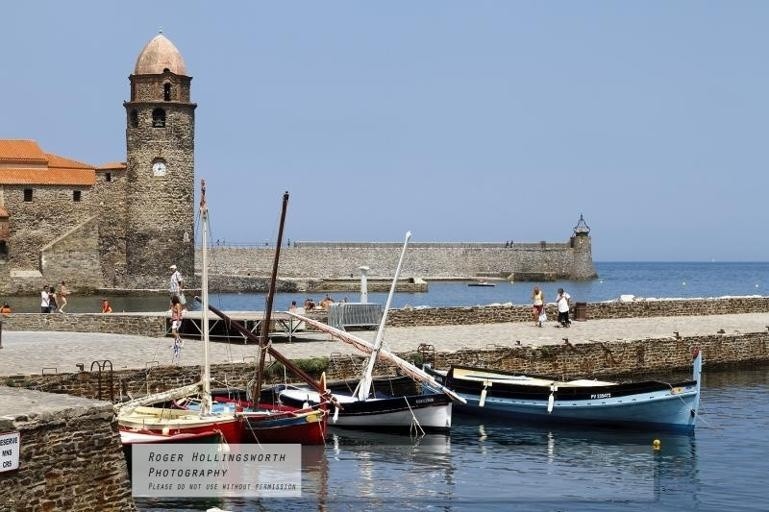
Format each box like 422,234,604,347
113,178,702,444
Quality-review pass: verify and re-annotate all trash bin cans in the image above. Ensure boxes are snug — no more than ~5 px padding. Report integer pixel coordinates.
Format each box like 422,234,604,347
575,302,587,321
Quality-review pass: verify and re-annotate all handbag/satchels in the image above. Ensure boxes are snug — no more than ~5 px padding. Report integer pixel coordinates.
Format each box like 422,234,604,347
539,314,547,322
178,295,186,305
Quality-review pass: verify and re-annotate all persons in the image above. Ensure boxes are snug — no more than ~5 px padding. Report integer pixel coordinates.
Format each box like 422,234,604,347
0,300,11,313
170,294,184,349
41,285,51,314
555,288,571,328
169,264,188,312
531,287,544,327
291,300,297,313
100,297,112,313
304,294,334,311
57,281,72,314
48,287,59,313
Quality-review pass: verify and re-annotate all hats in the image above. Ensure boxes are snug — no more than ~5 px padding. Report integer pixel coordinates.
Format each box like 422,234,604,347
170,265,177,268
